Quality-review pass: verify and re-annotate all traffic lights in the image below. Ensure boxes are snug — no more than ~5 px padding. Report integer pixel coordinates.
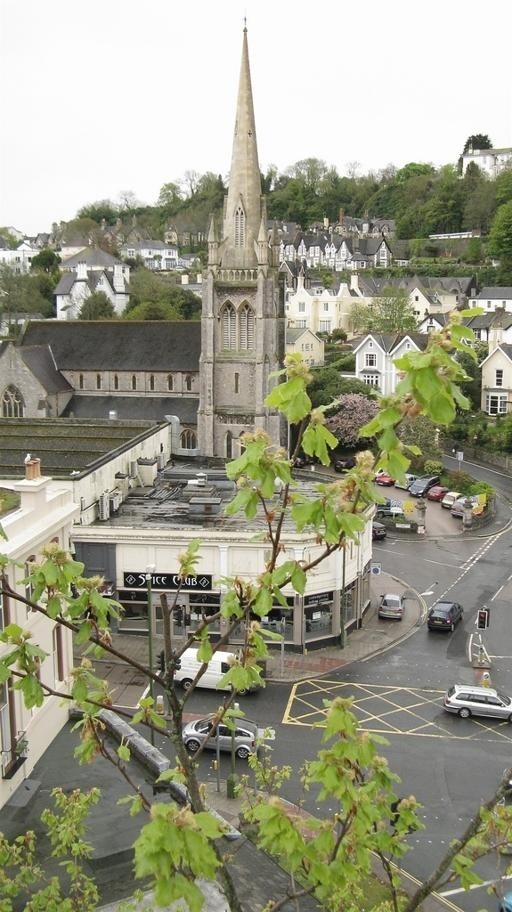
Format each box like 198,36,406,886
477,609,487,631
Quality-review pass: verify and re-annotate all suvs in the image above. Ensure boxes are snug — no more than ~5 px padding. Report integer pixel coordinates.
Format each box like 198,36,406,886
426,597,465,636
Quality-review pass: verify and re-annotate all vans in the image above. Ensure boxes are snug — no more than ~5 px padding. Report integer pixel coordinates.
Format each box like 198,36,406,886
172,646,262,697
442,684,512,722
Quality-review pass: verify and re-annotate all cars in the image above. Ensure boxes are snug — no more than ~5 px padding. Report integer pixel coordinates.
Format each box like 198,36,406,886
376,592,407,623
333,456,354,475
182,712,262,762
360,453,481,541
291,449,328,470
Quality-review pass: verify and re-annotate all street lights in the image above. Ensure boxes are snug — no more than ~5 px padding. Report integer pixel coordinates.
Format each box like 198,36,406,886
450,446,459,456
471,432,479,459
